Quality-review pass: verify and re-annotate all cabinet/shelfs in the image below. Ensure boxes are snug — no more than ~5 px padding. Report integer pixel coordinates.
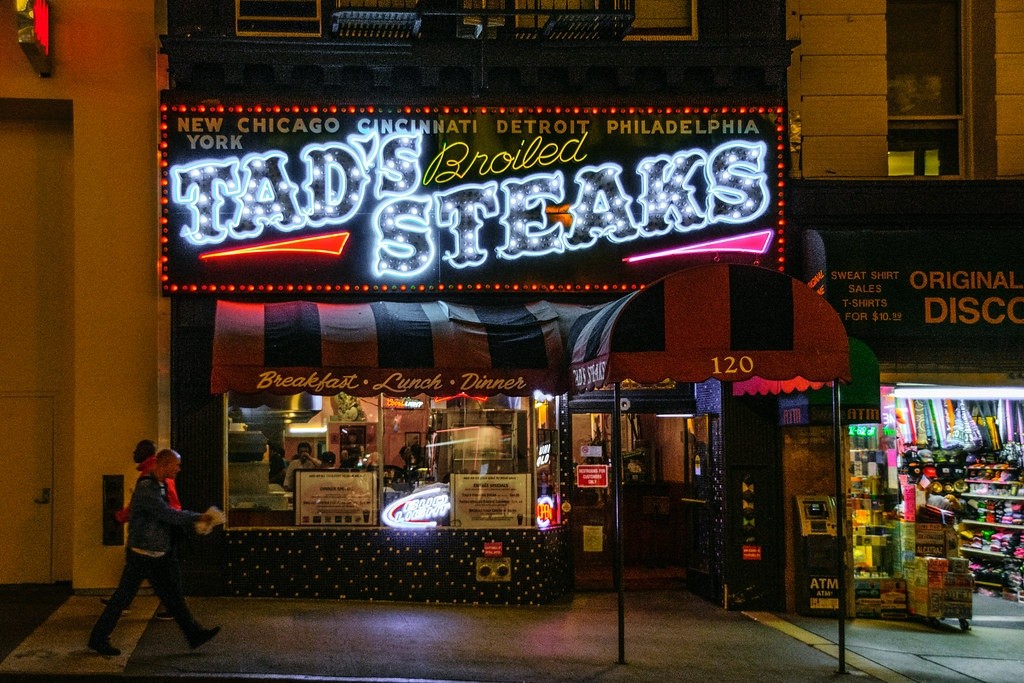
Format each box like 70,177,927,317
949,460,1024,601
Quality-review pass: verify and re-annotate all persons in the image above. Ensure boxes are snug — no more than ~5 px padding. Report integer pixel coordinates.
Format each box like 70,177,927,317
90,451,222,656
324,449,381,469
284,443,322,489
101,440,191,621
918,476,961,510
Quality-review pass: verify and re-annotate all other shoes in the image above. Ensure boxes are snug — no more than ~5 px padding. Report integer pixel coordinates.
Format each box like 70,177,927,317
87,637,121,656
155,608,174,619
191,626,221,649
99,597,129,613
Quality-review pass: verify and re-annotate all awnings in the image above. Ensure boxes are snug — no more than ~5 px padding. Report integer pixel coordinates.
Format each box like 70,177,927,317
209,263,851,675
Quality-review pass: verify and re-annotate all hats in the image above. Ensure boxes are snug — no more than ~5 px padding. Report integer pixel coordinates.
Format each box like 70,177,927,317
905,448,969,493
